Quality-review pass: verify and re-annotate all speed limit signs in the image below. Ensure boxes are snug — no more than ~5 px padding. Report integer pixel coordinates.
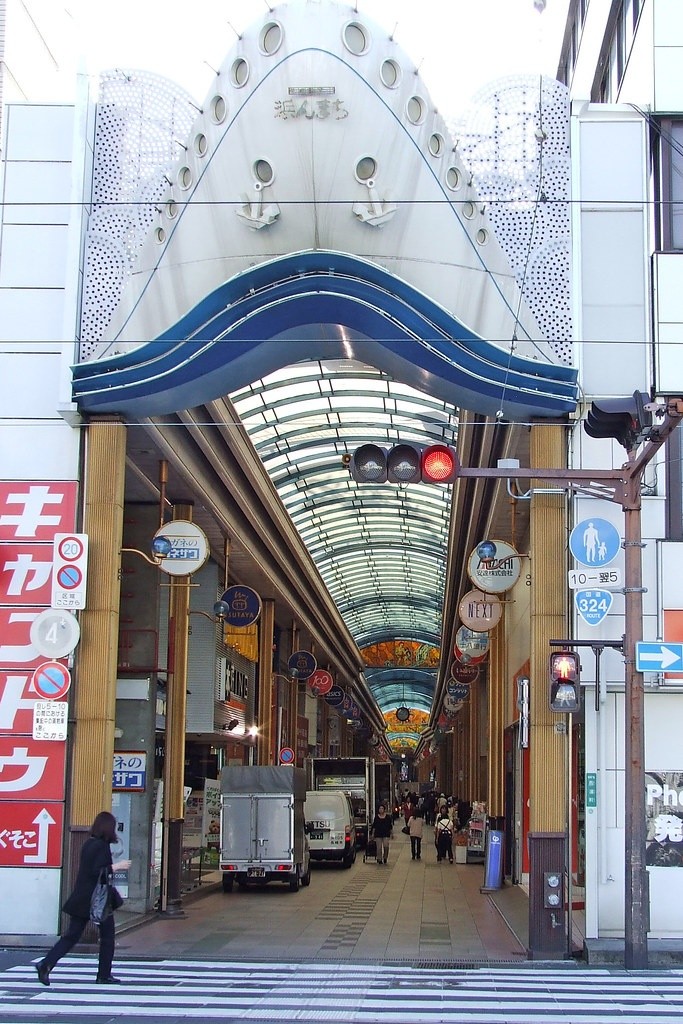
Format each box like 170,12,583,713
58,537,83,562
40,616,71,651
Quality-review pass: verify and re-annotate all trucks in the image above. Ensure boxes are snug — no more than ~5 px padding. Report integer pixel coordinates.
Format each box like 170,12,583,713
302,756,404,847
219,765,314,893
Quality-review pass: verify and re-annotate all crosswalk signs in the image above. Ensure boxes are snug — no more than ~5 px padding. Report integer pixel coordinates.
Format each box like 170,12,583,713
549,652,580,712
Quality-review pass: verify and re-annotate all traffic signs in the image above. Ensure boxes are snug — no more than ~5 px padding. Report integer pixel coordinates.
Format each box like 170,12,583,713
635,641,683,673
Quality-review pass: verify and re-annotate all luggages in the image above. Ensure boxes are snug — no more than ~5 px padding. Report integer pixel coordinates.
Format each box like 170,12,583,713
362,837,377,863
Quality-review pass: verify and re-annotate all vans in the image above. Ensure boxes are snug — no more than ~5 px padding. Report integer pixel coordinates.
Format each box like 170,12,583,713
303,790,359,869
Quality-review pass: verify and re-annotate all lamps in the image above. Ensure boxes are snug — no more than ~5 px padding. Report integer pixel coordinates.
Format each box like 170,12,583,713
460,653,490,667
477,540,533,570
393,683,413,725
186,600,229,623
272,668,298,684
122,536,172,566
298,686,321,698
223,719,239,732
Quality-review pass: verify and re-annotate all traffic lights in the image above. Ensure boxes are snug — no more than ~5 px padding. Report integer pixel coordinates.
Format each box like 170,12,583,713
348,443,459,484
584,390,652,448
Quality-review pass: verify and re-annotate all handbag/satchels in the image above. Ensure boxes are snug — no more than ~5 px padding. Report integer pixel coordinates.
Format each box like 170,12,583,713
106,869,124,910
89,867,109,925
402,826,410,835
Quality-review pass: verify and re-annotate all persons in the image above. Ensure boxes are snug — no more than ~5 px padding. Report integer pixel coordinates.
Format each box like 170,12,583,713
369,805,394,864
36,812,131,985
405,791,459,864
407,809,424,860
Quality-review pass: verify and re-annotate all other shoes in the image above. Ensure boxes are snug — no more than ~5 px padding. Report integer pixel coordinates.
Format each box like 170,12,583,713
412,856,415,859
438,860,441,863
450,859,453,863
379,861,382,864
35,960,50,985
417,855,420,858
96,976,120,983
384,858,387,863
